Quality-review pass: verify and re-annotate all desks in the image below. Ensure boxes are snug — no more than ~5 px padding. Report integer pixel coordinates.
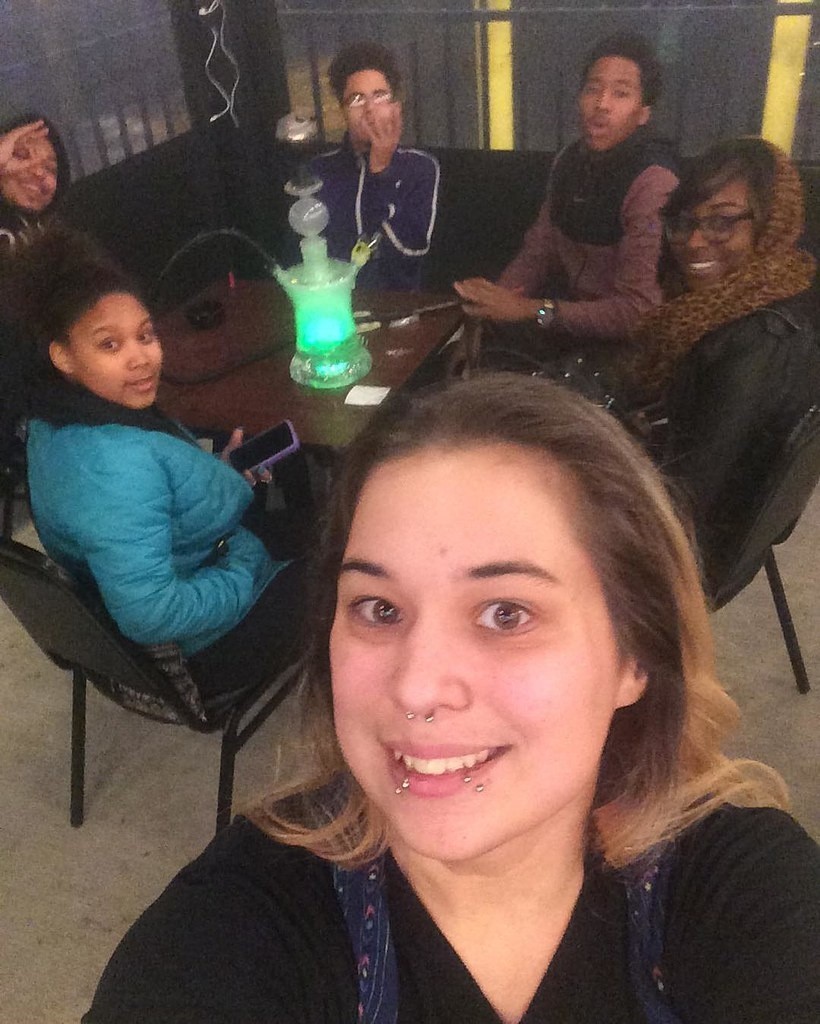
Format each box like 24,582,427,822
150,272,468,458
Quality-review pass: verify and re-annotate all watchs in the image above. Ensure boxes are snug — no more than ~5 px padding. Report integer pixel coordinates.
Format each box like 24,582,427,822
534,299,556,329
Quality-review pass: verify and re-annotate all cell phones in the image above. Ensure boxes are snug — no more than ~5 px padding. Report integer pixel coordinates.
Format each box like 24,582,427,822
229,418,300,473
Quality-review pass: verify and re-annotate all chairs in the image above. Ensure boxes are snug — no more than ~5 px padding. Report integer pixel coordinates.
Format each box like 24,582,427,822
0,400,820,838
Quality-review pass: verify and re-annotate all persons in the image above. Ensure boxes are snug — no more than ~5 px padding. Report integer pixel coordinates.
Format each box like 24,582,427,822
455,32,684,347
294,40,442,289
0,114,70,261
547,136,820,520
82,375,820,1024
0,225,324,696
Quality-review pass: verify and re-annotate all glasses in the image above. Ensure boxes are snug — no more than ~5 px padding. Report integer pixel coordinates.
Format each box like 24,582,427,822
661,212,753,245
343,90,393,109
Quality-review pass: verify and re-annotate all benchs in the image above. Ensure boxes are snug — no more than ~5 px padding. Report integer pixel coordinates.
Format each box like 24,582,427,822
0,126,820,540
